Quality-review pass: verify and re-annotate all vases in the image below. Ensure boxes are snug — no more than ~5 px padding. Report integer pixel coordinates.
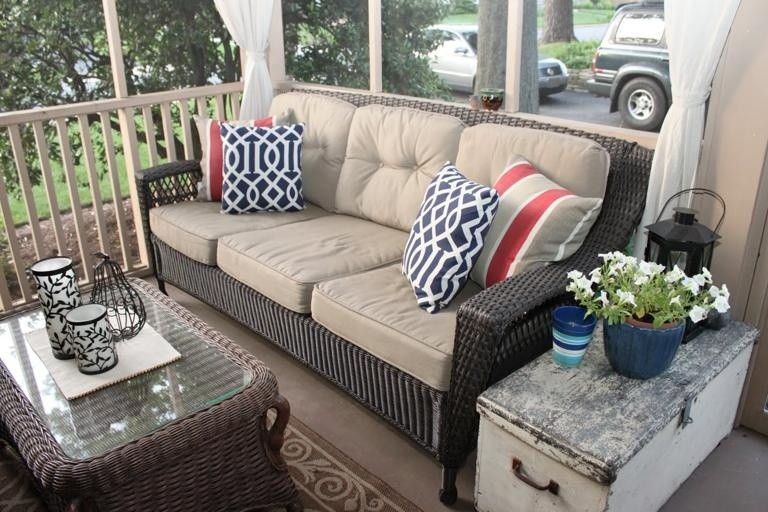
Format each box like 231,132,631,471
478,88,504,111
601,312,685,381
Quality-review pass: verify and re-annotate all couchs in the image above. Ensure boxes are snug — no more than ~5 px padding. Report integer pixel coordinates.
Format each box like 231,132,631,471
133,85,652,508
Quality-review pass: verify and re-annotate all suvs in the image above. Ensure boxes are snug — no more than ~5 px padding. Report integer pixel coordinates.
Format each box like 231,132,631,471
585,0,678,132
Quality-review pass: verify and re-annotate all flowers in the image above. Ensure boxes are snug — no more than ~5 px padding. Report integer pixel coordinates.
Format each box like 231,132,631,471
562,249,731,328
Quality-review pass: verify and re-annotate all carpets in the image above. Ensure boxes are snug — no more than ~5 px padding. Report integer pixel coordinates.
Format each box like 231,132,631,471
0,402,433,512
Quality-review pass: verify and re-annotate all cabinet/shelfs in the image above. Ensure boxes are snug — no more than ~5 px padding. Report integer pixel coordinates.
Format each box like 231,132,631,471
473,317,760,512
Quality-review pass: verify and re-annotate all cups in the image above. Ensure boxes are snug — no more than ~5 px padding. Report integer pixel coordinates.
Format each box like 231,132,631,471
551,305,597,369
31,255,88,360
65,303,118,374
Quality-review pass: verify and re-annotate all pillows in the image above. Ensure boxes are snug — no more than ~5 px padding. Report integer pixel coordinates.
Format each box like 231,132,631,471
214,120,306,214
466,152,604,289
400,160,499,315
189,106,291,201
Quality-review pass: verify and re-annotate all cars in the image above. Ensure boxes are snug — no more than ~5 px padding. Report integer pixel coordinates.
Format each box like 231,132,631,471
7,38,224,116
408,23,571,102
